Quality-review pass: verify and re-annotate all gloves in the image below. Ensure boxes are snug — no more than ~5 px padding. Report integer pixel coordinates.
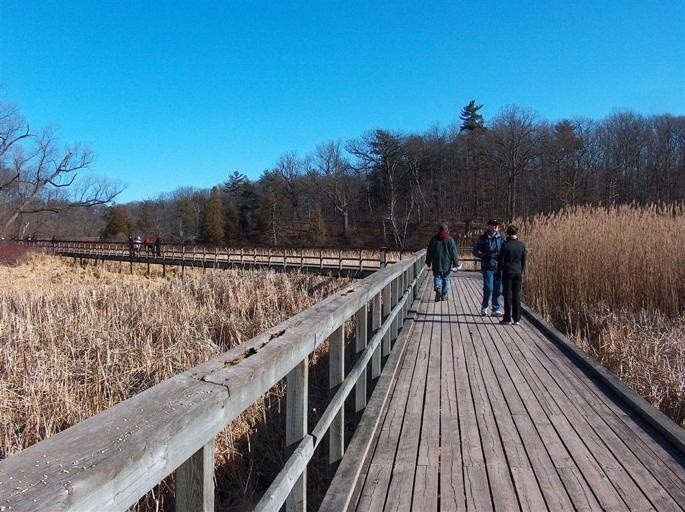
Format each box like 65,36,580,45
482,252,491,258
496,272,501,280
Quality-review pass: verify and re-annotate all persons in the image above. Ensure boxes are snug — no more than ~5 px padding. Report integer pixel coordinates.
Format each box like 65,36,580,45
425,222,459,302
127,232,161,257
496,224,527,325
26,231,38,248
50,234,58,244
472,217,506,317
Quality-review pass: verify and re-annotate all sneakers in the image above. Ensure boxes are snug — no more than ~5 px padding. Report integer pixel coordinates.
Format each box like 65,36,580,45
442,295,448,301
500,320,520,325
435,289,441,302
491,311,504,317
481,307,487,316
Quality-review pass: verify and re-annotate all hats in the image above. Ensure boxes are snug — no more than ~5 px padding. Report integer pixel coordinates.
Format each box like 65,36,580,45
488,219,500,225
